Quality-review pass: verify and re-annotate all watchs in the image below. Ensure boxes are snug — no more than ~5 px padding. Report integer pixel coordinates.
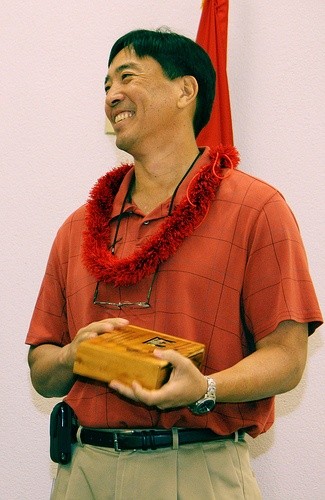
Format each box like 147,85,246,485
188,375,216,415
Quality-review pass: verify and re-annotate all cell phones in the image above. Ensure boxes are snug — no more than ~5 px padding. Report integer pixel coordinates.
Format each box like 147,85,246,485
57,406,66,463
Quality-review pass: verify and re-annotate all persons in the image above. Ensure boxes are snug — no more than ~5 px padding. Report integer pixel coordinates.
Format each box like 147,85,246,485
25,23,323,500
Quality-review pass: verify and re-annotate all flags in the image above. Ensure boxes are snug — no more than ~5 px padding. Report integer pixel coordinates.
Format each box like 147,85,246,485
193,0,242,171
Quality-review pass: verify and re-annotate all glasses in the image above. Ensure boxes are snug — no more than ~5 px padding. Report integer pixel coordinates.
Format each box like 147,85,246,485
92,243,160,311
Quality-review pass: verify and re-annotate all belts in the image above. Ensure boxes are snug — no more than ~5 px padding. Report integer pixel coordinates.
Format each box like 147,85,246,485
72,425,246,453
